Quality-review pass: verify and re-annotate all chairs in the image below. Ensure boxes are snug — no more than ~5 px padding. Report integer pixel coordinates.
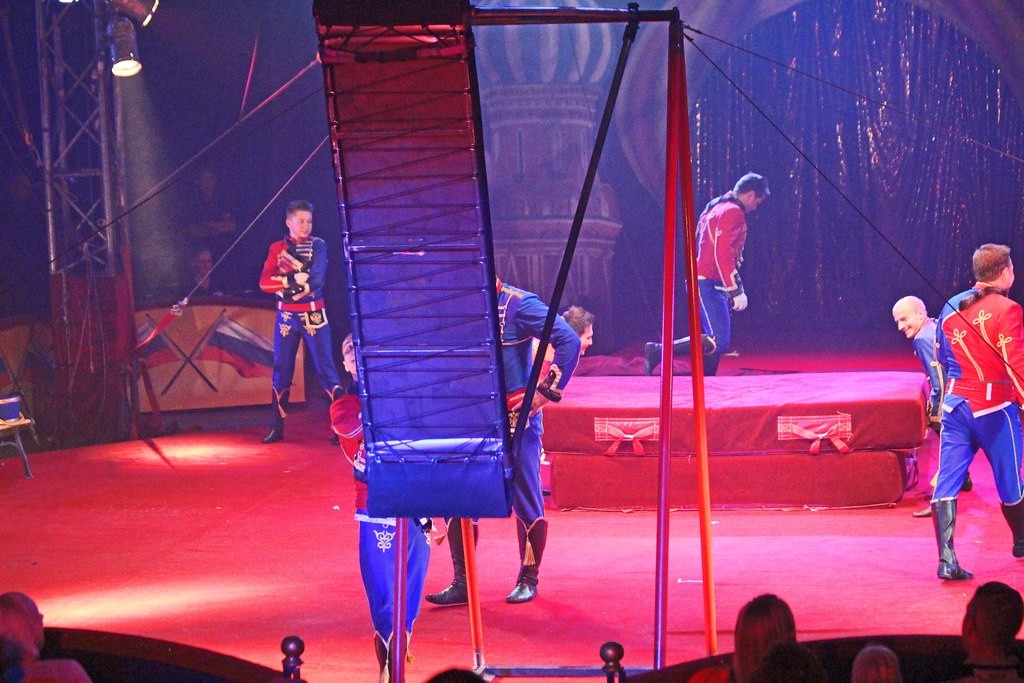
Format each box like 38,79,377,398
0,348,37,481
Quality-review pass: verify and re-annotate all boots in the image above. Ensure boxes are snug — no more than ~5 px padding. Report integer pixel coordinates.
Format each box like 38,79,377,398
930,497,973,579
262,387,290,442
999,488,1024,558
645,334,719,376
425,518,479,606
373,630,413,683
506,514,548,603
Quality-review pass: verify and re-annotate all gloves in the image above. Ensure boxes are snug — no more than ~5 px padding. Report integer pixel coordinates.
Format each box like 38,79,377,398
732,292,747,312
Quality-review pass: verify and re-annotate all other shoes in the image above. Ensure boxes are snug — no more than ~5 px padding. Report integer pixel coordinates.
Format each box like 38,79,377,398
913,506,932,517
323,385,345,445
959,477,972,491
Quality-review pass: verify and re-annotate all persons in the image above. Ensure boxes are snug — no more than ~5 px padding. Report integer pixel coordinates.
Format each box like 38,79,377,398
931,242,1024,580
893,296,972,491
530,305,593,384
645,173,771,376
851,644,903,682
424,274,581,605
17,324,58,430
0,591,91,683
960,582,1024,683
258,199,344,446
184,168,236,246
734,594,796,682
186,247,223,296
751,641,829,683
329,330,431,683
